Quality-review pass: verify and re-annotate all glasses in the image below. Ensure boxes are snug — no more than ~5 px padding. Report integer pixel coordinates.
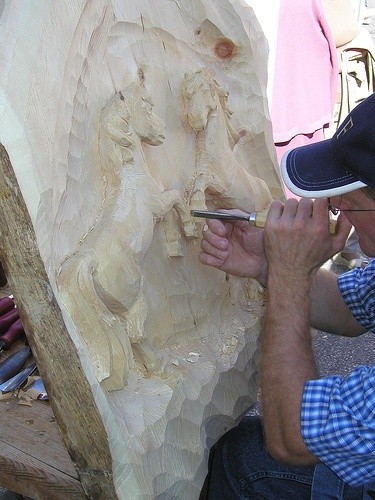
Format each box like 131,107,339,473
328,197,375,215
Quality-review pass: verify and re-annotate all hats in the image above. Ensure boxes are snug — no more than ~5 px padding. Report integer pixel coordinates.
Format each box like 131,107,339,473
280,92,375,198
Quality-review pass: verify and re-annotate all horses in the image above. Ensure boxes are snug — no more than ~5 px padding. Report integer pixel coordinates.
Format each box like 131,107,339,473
56,81,199,394
180,68,275,303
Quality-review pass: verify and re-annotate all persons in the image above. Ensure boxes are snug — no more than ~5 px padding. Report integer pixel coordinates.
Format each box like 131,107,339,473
199,93,375,500
